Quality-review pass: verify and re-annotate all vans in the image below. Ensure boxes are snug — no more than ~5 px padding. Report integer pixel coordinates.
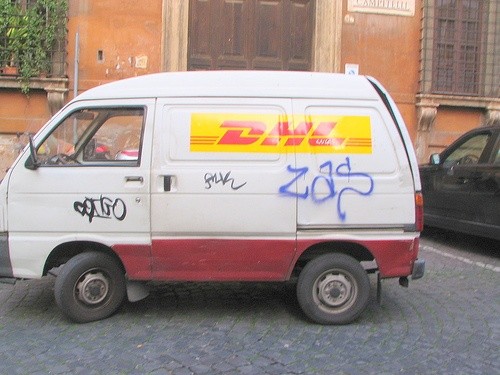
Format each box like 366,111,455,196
0,70,425,326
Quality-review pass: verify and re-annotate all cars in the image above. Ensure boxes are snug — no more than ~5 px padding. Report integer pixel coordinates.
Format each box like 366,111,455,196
419,124,500,246
